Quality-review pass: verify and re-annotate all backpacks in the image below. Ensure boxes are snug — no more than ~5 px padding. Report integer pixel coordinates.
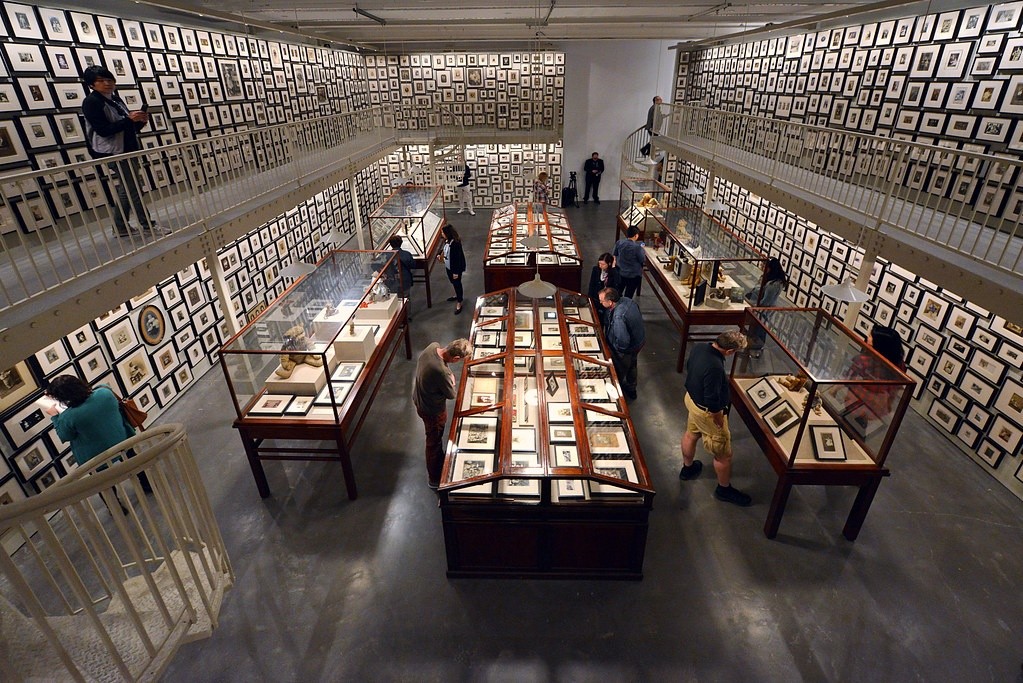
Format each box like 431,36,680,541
562,187,572,208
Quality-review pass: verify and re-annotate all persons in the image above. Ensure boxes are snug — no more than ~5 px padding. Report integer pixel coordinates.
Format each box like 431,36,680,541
44,374,157,516
80,64,173,235
412,336,473,490
436,223,466,315
371,234,416,329
451,154,476,216
598,288,646,400
680,330,753,507
748,256,788,357
533,171,550,202
584,151,605,205
610,225,646,299
403,182,419,216
831,325,907,446
588,252,620,332
639,96,669,156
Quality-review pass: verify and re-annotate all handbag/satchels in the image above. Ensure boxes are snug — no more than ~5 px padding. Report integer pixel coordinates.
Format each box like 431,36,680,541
118,398,147,428
411,273,413,286
439,250,445,264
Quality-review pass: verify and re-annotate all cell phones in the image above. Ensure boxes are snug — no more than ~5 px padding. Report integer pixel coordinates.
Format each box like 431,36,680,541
141,104,148,113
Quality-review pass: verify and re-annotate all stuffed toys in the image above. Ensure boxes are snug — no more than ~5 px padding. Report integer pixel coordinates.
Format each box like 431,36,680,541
661,255,726,289
648,198,661,212
274,323,323,379
675,218,692,239
637,192,651,207
800,389,822,415
776,366,810,391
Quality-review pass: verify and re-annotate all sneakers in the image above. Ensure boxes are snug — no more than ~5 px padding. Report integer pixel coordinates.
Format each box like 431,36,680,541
679,459,702,480
714,482,752,506
143,224,172,236
112,226,139,238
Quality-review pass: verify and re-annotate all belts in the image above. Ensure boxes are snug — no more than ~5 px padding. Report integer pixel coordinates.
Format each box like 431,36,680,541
687,390,709,412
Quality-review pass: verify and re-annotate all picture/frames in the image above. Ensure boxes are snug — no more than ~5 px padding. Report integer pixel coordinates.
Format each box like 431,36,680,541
2,0,366,546
451,284,642,504
643,1,1023,505
370,50,584,268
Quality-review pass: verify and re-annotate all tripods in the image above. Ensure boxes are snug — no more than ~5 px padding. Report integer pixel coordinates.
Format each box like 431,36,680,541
568,171,579,208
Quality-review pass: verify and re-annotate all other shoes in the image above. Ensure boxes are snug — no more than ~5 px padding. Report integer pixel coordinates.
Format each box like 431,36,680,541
428,481,439,489
398,326,404,331
469,210,475,216
141,484,153,493
407,317,412,322
457,208,464,213
594,199,600,203
447,297,457,301
640,149,646,156
109,506,128,516
454,305,462,314
584,200,588,204
646,152,649,155
625,387,637,399
